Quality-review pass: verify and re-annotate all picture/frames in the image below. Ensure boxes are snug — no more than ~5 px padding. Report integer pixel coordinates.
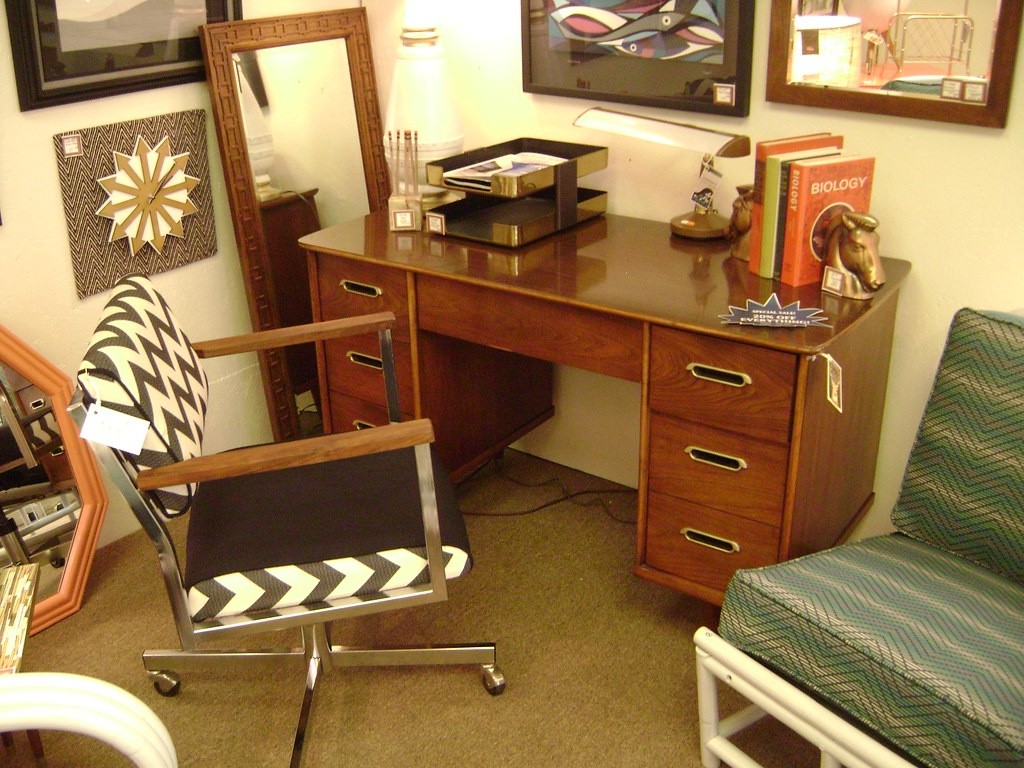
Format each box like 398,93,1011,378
798,0,839,17
520,0,755,118
3,0,245,111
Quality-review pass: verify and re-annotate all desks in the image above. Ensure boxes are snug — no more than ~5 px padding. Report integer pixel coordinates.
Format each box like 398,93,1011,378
0,561,41,675
298,191,914,614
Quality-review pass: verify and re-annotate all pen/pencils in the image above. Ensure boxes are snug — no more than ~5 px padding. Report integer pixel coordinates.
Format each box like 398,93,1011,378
383,129,424,195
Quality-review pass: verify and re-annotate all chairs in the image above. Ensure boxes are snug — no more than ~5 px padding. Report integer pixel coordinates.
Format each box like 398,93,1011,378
67,269,506,768
0,425,77,569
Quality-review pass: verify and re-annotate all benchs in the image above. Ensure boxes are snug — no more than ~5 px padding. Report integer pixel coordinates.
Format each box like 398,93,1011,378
693,307,1024,768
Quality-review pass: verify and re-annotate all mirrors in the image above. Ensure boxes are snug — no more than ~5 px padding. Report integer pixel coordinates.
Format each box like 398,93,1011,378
199,8,399,447
765,0,1024,129
0,323,110,639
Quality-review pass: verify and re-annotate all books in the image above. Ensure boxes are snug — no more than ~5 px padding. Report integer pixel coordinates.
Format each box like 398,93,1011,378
747,132,877,287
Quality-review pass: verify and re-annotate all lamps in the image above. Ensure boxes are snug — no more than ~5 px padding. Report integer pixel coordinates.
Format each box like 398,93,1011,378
572,103,752,239
